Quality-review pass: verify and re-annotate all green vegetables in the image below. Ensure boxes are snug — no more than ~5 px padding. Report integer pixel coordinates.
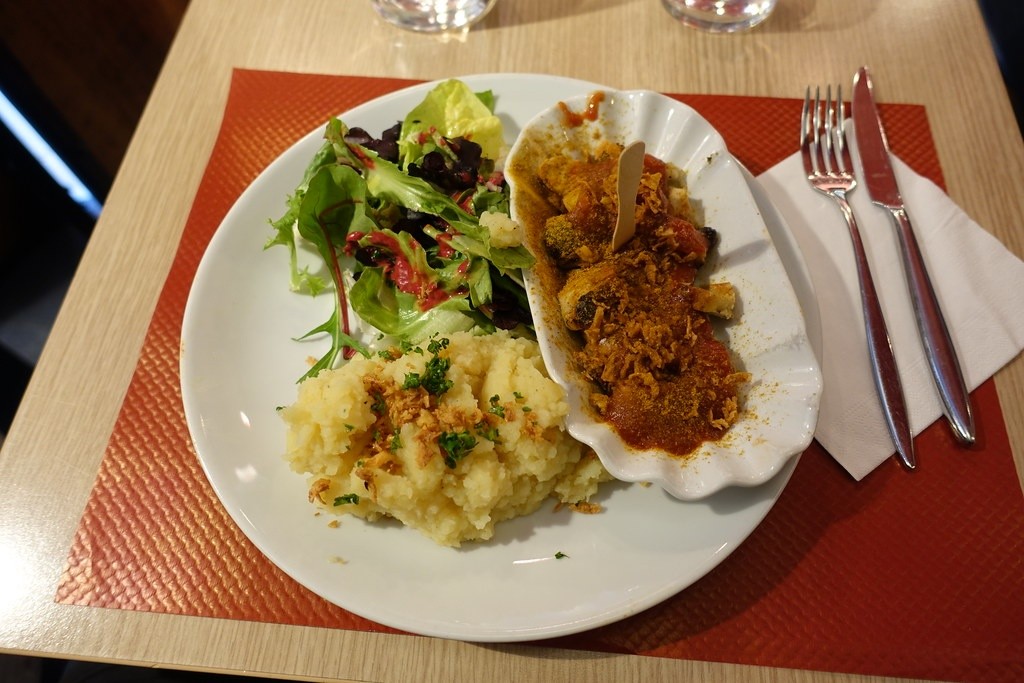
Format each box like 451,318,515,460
260,77,538,384
274,332,531,507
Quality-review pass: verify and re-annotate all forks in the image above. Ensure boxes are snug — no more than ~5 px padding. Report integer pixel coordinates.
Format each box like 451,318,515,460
800,85,913,467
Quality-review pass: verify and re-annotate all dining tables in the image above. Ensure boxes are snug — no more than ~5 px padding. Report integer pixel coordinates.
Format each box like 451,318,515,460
747,116,1023,483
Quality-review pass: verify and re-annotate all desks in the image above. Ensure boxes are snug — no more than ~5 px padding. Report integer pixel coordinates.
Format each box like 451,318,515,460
0,0,1024,683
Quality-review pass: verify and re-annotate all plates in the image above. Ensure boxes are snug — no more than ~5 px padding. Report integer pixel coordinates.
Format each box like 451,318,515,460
506,90,822,502
179,74,800,643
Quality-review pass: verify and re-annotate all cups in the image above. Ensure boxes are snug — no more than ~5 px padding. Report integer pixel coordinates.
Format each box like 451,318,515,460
661,0,776,34
370,0,496,33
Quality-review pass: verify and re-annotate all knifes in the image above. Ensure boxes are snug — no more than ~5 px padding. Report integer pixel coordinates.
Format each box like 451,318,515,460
853,66,975,447
